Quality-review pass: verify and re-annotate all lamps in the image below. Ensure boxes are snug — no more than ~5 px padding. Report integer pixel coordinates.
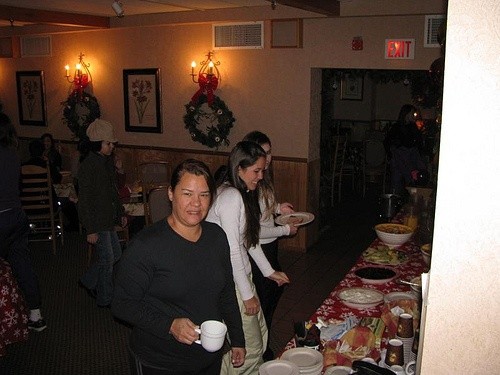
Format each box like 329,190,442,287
64,54,88,91
112,2,126,17
190,54,225,97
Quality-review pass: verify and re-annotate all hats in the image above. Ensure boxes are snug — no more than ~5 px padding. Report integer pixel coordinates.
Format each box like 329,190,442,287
86,119,118,143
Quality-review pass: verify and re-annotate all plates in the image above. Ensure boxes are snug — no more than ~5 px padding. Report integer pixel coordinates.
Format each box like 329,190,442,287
275,212,315,226
365,249,407,266
326,366,352,375
385,292,416,304
410,276,422,293
395,264,428,286
259,347,323,375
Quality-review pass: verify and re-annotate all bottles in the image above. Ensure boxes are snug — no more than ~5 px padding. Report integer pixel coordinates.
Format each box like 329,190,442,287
404,194,435,243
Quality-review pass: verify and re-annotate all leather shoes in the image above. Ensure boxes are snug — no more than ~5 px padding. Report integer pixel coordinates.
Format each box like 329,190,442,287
97,304,111,309
78,279,97,298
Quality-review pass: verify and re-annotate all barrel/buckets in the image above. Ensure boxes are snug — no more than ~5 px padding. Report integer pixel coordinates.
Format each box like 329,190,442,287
378,193,402,222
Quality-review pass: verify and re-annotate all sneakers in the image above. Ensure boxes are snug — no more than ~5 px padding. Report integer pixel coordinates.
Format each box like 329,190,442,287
27,317,47,332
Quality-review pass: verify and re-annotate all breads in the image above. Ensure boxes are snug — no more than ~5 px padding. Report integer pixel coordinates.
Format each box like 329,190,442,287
322,352,352,366
341,325,376,350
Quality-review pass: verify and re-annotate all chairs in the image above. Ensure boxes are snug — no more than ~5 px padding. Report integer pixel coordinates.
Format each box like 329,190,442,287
88,160,176,261
321,133,383,203
20,157,64,255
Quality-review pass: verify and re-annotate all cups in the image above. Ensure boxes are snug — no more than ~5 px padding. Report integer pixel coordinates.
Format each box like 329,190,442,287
195,320,227,352
385,313,420,375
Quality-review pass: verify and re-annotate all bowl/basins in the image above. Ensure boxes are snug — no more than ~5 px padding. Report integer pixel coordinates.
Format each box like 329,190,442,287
354,265,401,284
374,223,414,245
335,287,385,309
421,244,432,265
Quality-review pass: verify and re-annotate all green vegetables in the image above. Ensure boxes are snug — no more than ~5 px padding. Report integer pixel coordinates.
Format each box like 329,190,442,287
369,250,406,263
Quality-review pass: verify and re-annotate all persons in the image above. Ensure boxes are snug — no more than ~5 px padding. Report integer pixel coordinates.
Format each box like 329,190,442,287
112,159,247,375
243,131,298,362
77,119,140,308
20,139,62,215
40,134,62,171
383,105,424,194
204,142,290,375
0,113,48,332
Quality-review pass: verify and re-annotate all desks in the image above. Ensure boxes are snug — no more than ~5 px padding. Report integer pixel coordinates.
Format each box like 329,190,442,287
122,183,166,217
53,170,77,204
270,196,433,375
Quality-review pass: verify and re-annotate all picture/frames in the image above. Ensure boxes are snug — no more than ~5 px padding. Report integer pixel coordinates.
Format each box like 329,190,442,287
16,70,47,128
338,75,365,100
122,68,163,132
